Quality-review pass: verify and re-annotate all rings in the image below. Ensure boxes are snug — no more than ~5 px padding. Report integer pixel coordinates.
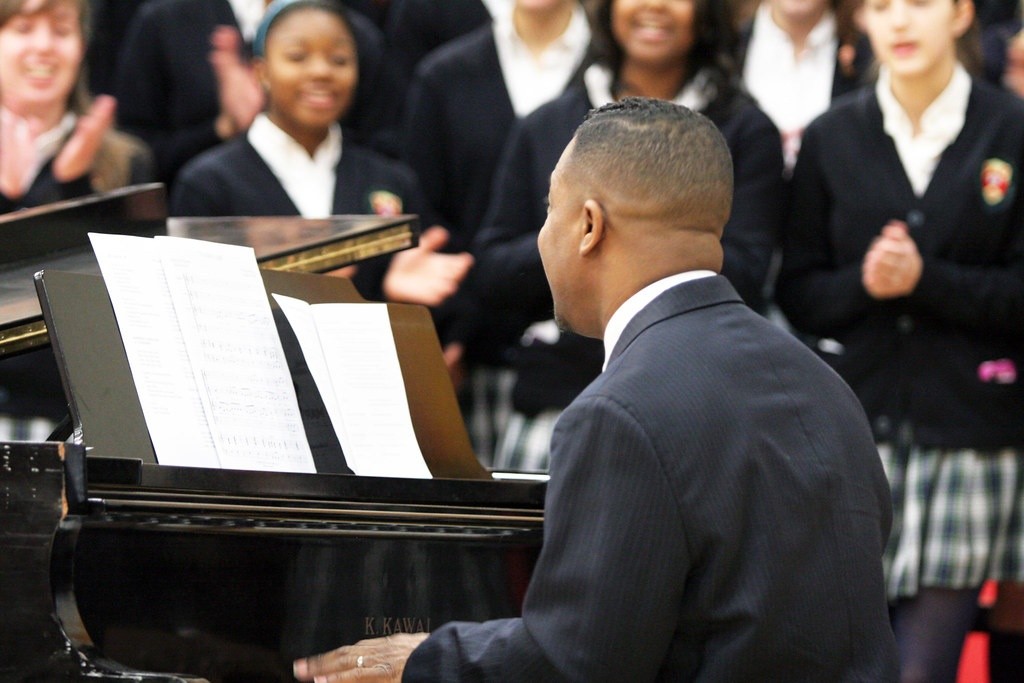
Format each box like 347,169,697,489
357,654,364,668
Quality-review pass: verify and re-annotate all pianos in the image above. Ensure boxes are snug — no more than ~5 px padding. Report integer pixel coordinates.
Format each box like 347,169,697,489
1,272,557,683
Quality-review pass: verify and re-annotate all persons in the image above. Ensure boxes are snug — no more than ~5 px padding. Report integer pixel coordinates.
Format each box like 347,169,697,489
1,0,1023,682
293,98,911,682
